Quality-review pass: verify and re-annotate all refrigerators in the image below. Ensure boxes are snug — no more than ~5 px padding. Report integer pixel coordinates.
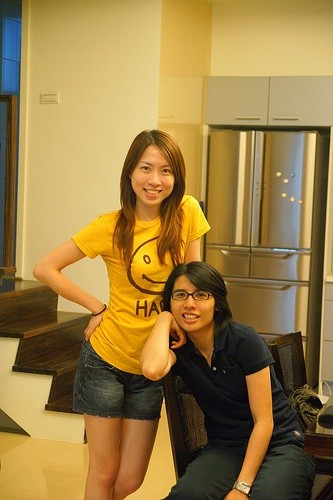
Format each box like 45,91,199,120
205,127,329,401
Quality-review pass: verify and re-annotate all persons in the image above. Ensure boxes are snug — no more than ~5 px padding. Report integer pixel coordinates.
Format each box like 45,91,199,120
33,129,212,500
141,261,315,500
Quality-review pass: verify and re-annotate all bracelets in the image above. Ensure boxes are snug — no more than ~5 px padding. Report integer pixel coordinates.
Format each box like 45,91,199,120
92,304,107,316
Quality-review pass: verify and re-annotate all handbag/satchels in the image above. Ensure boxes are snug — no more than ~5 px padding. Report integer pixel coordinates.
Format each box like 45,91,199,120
288,381,322,427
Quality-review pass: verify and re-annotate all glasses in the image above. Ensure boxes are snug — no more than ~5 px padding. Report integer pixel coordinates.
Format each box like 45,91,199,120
170,290,212,301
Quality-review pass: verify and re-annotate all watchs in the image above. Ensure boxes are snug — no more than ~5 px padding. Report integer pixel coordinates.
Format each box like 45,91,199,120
232,481,251,495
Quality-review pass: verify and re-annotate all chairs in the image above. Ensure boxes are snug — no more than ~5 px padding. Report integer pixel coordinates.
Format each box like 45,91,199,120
267,331,307,432
162,332,333,500
